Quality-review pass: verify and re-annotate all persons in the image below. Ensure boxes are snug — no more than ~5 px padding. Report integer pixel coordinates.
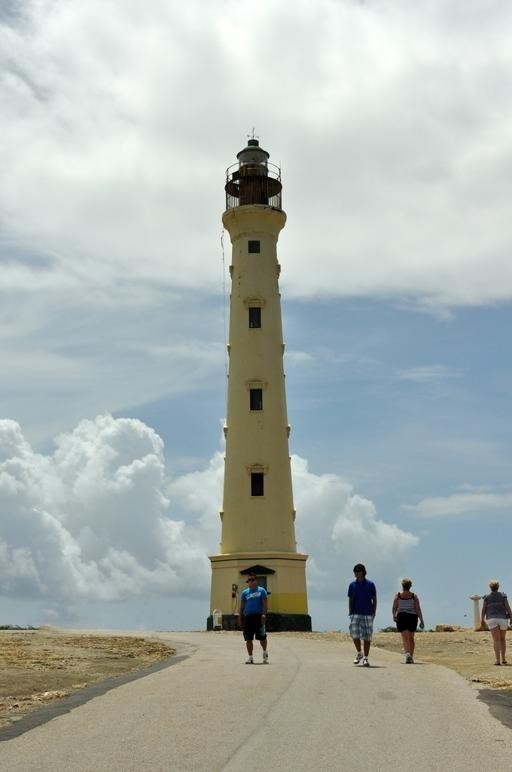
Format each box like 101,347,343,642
392,576,425,664
347,563,378,667
239,573,270,664
480,580,512,665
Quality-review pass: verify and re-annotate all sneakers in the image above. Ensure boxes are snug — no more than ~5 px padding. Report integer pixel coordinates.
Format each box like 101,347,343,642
245,658,254,664
406,656,414,664
363,659,369,667
263,653,269,664
353,654,363,664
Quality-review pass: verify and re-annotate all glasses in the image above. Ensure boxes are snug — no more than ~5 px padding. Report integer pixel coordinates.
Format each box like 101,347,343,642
248,578,256,582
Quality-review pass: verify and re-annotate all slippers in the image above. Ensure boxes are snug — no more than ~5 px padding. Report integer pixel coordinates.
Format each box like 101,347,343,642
493,661,507,665
